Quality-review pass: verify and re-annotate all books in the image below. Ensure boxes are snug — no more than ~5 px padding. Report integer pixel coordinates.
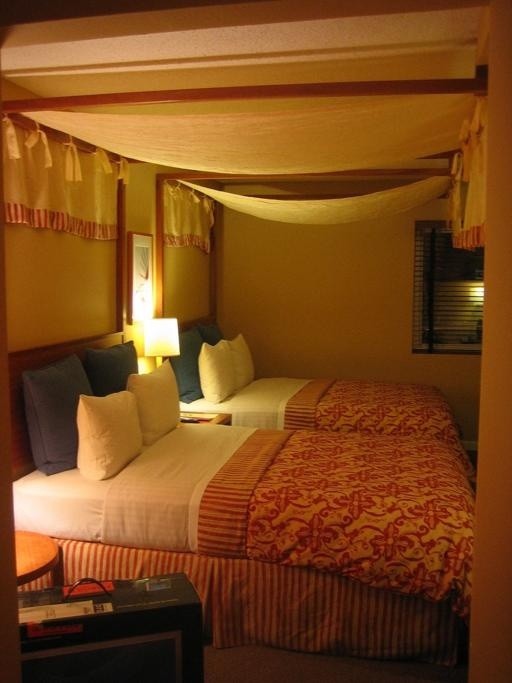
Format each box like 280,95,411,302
62,581,115,598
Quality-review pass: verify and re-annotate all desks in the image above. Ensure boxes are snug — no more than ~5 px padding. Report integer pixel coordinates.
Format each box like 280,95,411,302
14,530,61,586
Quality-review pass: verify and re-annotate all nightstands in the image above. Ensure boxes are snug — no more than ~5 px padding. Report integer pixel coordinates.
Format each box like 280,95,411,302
178,410,232,426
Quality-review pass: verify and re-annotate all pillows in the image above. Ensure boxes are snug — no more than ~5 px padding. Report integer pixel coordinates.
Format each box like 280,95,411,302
77,390,143,481
127,358,179,443
198,339,237,404
170,325,206,404
82,339,139,397
19,353,99,477
197,320,223,346
226,332,256,390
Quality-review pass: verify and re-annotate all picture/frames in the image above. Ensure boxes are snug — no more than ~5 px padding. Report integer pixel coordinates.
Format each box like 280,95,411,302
127,229,154,325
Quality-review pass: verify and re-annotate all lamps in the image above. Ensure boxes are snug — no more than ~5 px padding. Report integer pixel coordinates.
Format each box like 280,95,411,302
143,317,181,369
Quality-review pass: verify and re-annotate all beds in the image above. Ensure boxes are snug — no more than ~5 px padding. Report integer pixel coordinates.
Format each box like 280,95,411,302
154,165,475,480
0,59,488,682
